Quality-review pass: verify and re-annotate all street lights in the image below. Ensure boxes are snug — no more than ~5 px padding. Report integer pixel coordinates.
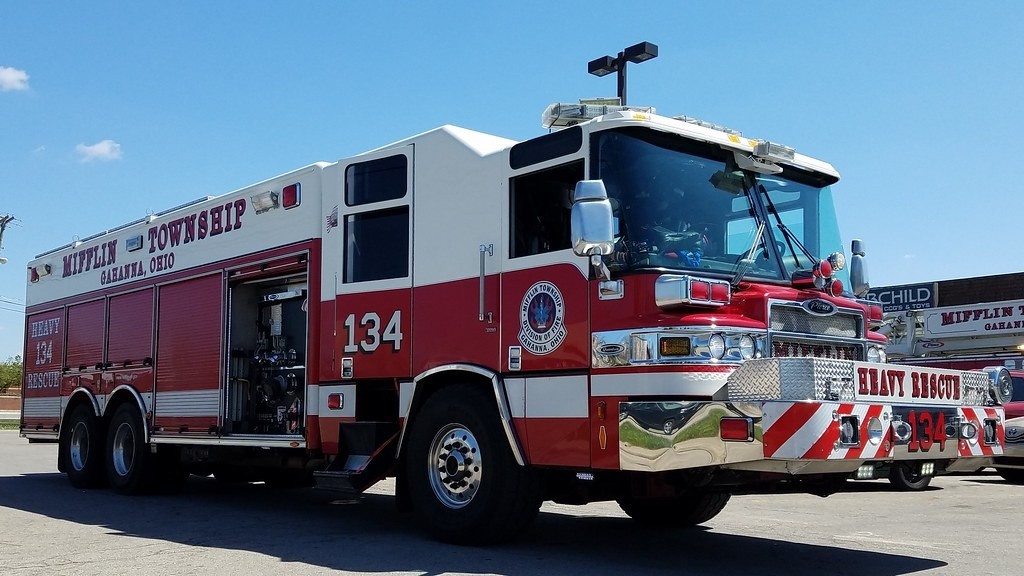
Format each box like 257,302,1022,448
588,40,659,107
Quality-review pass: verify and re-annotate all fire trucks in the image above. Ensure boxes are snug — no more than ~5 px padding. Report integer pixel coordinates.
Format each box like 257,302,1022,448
803,350,1023,497
19,104,1013,543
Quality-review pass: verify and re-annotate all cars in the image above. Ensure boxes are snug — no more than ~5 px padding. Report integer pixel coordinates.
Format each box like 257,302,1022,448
970,369,1024,420
982,416,1024,484
